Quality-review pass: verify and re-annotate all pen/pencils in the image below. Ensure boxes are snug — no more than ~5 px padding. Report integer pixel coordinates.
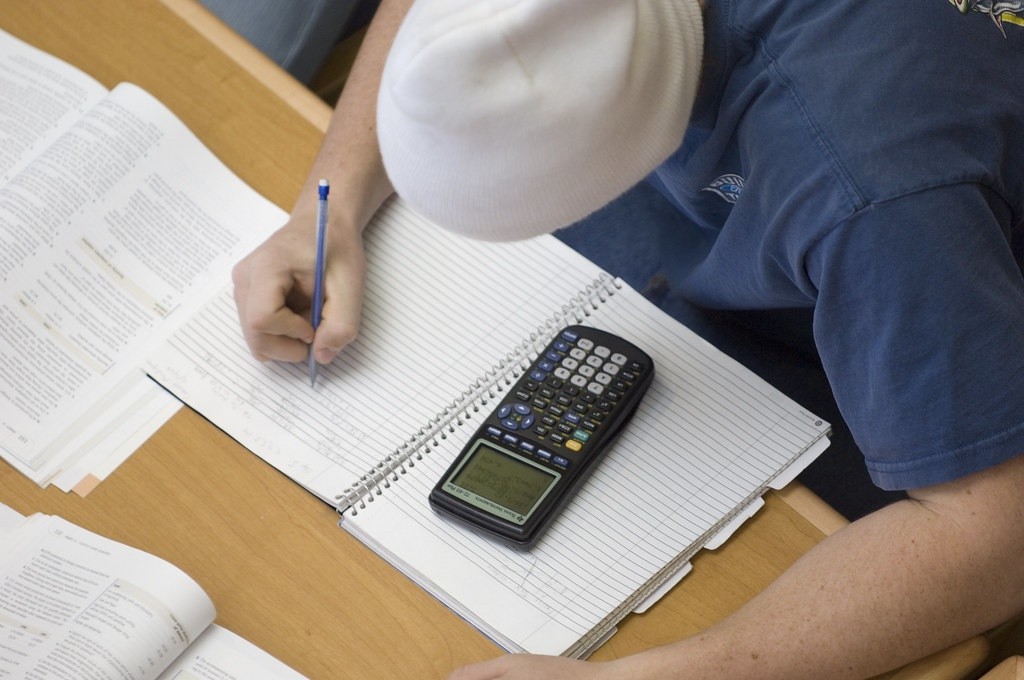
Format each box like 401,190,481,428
308,178,332,391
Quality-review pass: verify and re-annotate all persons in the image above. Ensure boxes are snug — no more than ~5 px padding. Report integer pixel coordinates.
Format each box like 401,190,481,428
200,1,356,87
233,0,1024,680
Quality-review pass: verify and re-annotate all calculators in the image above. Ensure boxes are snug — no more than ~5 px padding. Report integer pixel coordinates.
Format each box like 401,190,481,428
427,325,656,553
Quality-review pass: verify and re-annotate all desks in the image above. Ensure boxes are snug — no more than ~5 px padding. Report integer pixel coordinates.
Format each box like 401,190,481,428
0,1,993,680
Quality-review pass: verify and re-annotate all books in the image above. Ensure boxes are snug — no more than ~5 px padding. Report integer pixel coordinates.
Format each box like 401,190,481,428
1,26,828,680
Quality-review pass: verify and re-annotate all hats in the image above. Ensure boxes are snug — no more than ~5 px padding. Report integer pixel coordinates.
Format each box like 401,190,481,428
376,0,704,243
